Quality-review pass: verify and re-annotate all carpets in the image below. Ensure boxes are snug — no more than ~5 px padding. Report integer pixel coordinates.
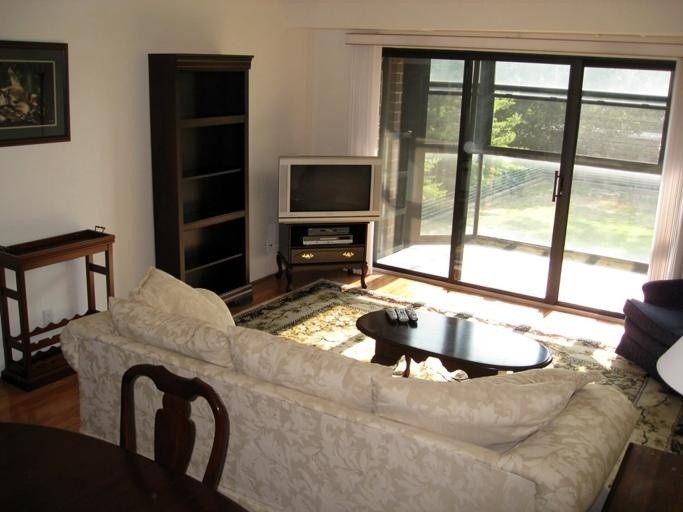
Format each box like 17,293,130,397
228,278,681,453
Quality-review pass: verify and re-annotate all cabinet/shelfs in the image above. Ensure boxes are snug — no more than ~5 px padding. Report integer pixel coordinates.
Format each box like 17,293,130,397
0,226,115,391
148,53,254,308
276,222,370,289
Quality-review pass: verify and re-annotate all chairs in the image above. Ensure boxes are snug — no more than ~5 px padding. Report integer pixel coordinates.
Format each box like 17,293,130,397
120,364,230,491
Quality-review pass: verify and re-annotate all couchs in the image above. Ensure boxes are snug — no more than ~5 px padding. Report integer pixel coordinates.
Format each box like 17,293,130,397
59,265,640,511
615,278,682,398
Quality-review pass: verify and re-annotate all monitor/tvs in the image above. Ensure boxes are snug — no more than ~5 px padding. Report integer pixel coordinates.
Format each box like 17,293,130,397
277,154,385,224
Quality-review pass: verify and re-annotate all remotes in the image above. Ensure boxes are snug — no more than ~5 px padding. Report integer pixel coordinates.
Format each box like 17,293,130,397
398,306,407,322
383,306,396,322
406,304,419,322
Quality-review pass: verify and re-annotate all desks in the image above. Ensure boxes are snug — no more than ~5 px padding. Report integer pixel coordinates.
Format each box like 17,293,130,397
0,422,250,511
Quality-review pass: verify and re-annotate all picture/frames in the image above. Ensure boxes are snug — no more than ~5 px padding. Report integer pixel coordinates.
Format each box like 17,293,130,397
2,40,69,146
